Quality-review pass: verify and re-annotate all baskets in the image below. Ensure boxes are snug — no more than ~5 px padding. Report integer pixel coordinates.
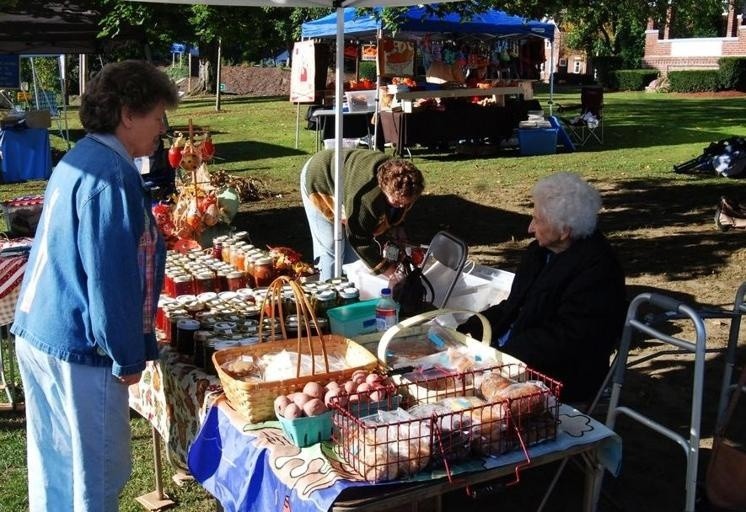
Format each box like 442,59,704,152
211,276,378,423
351,307,528,411
330,365,564,484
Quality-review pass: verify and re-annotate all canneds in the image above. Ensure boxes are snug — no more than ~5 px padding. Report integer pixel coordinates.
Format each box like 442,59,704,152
154,230,359,375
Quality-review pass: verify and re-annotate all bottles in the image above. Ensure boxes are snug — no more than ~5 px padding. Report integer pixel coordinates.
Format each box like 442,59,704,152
161,235,271,294
374,288,398,330
158,281,360,375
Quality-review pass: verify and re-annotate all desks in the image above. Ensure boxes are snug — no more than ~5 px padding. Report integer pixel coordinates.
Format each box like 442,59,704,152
0,195,607,512
0,128,51,182
307,85,543,161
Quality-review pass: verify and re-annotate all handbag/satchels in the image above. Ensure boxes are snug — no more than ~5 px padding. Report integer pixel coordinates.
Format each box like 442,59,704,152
704,436,745,508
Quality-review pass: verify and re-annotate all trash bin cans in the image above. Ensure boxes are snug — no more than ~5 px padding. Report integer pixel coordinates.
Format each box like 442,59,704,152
579,85,604,120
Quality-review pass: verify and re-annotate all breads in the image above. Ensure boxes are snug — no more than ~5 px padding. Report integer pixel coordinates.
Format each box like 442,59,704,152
218,342,553,478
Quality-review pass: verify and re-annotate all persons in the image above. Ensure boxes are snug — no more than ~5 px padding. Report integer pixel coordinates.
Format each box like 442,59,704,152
455,170,624,406
300,148,424,283
9,60,181,512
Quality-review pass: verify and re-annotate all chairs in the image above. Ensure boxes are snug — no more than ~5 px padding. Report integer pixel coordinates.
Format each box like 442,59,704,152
559,87,604,147
536,298,640,511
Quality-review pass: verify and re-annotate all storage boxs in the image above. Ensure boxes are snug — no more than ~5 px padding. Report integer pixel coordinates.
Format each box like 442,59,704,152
517,127,558,154
